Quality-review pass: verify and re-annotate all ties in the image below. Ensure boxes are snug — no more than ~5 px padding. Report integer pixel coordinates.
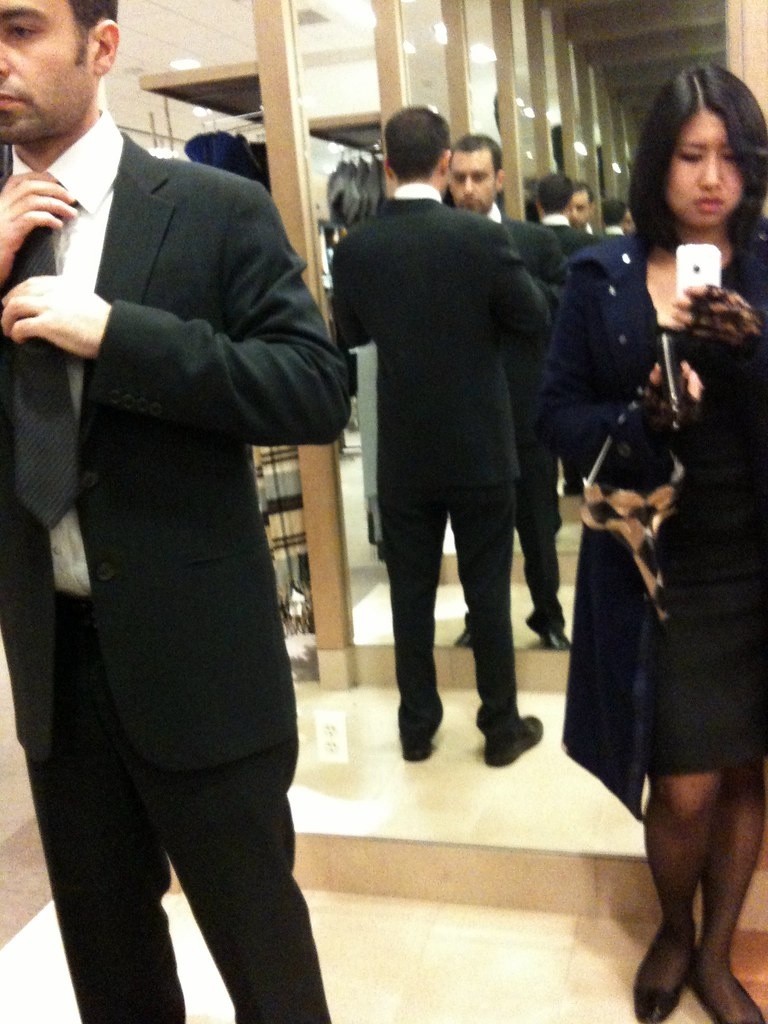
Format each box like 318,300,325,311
0,226,80,529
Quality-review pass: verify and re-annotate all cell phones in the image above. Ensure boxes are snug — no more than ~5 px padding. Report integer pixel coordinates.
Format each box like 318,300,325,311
675,243,722,306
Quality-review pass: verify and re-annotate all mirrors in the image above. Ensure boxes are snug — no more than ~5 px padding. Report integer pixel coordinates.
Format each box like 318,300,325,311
286,0,768,652
0,0,768,1023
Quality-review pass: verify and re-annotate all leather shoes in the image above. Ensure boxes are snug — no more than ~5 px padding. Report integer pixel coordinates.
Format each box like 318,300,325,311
541,626,570,651
456,631,470,647
485,716,543,768
403,742,431,761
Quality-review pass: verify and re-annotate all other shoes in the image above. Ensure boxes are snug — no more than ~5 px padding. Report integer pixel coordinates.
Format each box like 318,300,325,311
689,971,766,1024
633,946,695,1024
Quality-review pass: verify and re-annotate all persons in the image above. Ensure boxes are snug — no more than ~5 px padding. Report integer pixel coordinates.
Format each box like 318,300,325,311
446,134,572,656
600,197,637,238
1,0,353,1024
530,173,601,495
546,64,766,1024
330,106,553,768
